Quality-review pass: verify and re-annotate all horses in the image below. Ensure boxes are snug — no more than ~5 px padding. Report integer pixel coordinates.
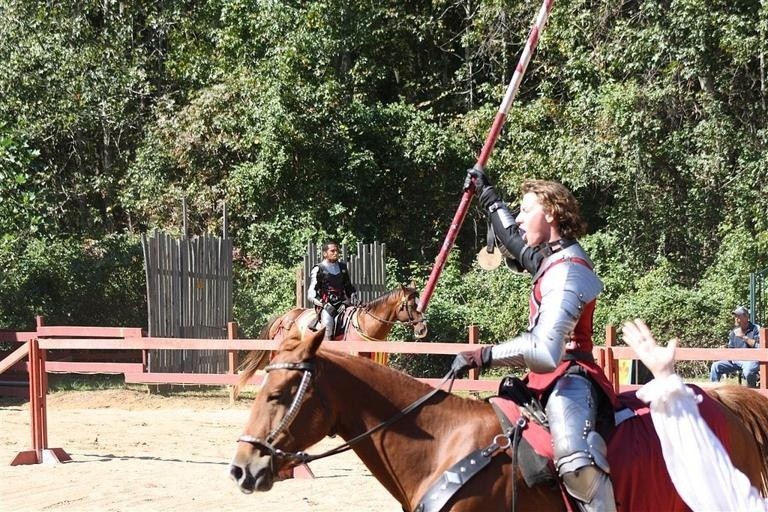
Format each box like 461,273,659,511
228,326,768,512
229,280,428,403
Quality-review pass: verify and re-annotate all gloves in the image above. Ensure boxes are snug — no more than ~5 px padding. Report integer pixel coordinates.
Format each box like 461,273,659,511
463,168,502,212
323,302,339,317
451,345,494,379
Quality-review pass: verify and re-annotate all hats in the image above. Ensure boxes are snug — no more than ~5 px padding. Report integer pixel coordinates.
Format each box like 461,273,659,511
731,306,750,317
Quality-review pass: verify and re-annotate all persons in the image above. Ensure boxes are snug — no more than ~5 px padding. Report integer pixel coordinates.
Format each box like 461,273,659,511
621,318,768,512
307,240,360,341
711,306,762,388
451,168,618,512
